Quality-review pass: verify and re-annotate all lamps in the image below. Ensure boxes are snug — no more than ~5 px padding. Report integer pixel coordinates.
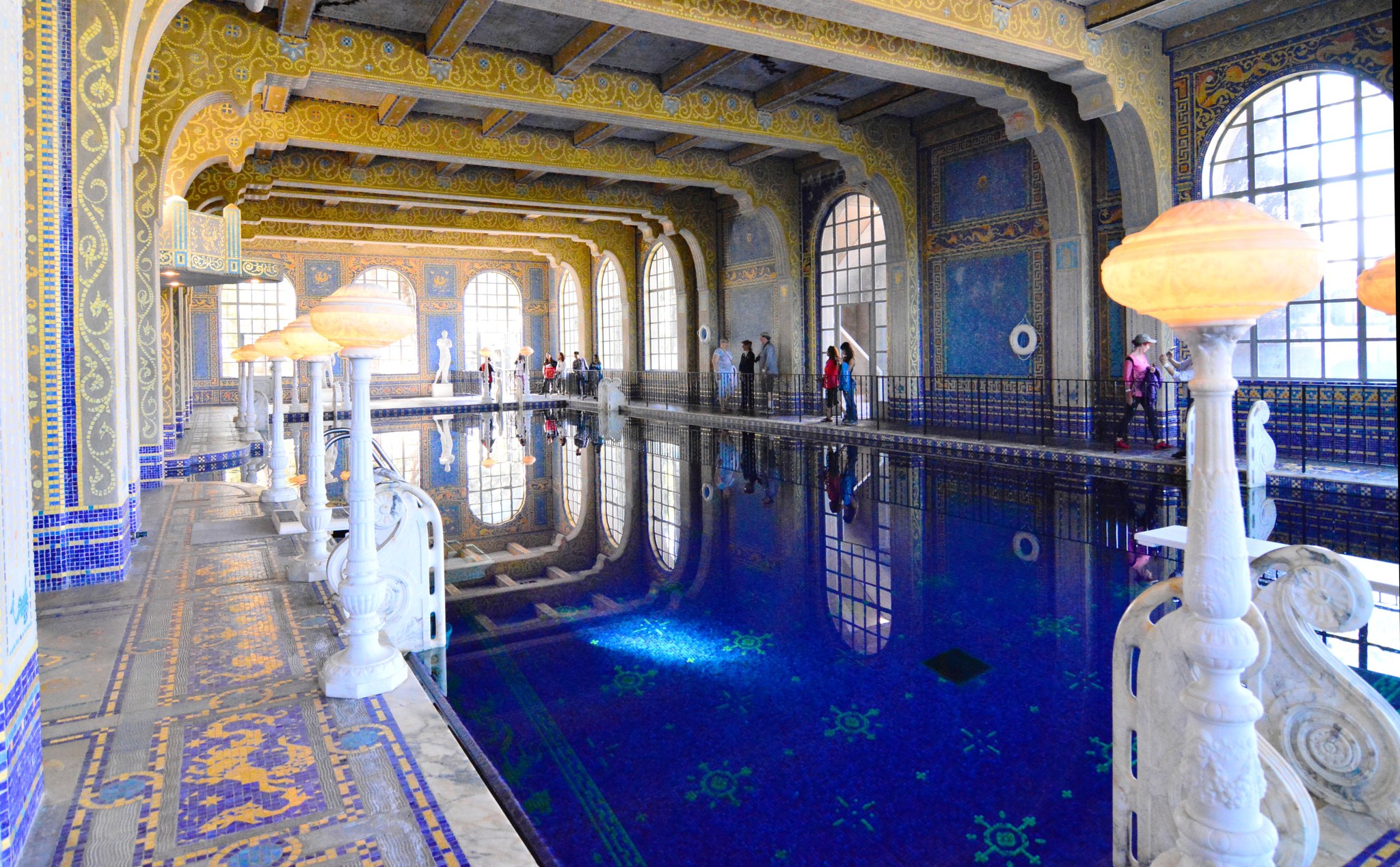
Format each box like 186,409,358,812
1100,192,1325,324
520,347,534,356
231,278,419,361
1353,253,1397,317
479,347,494,357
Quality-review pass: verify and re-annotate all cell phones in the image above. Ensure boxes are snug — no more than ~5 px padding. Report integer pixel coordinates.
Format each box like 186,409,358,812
834,354,839,361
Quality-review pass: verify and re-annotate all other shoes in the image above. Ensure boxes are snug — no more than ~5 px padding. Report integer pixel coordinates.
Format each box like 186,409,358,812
737,407,747,411
720,408,733,413
1171,449,1186,457
523,391,524,394
842,419,849,425
492,398,494,400
845,419,858,426
747,407,754,413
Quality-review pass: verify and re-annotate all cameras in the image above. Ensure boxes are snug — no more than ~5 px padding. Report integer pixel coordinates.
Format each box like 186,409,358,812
755,356,761,362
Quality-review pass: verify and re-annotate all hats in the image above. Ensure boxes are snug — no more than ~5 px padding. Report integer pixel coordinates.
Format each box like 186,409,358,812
760,332,772,339
1134,333,1157,344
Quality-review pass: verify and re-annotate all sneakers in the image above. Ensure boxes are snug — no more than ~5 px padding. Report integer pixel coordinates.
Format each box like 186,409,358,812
539,392,544,395
583,395,587,398
1116,439,1131,449
561,392,565,395
577,396,581,399
593,396,597,399
557,392,561,395
758,408,768,413
549,392,552,395
765,408,775,414
1154,441,1174,450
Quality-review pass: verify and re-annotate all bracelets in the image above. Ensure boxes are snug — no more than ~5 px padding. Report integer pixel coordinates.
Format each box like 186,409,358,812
1127,531,1132,534
764,470,767,473
1163,363,1169,369
762,369,766,372
1125,389,1131,392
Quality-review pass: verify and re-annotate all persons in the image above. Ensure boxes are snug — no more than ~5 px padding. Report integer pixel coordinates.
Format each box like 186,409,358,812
538,352,558,395
1159,351,1195,459
713,337,734,413
556,408,568,446
572,351,589,399
515,414,526,446
574,410,590,456
322,353,336,388
542,409,559,445
1115,479,1162,588
820,345,846,422
323,425,338,480
1116,333,1175,449
555,352,566,395
434,419,455,472
589,354,603,402
839,445,858,524
834,342,858,425
759,434,779,504
589,412,603,454
514,353,526,394
737,340,755,416
758,332,778,414
713,429,737,499
823,442,847,513
480,418,496,453
741,431,757,494
479,357,495,400
432,330,453,384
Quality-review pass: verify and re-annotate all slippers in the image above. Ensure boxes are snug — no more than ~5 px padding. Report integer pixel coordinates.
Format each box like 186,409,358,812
821,417,833,422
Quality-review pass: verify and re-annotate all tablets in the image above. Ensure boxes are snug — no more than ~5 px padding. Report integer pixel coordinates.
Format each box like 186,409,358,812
1165,346,1178,358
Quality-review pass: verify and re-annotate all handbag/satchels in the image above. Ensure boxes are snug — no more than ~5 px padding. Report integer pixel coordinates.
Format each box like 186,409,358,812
553,373,559,378
577,375,584,380
546,367,555,379
